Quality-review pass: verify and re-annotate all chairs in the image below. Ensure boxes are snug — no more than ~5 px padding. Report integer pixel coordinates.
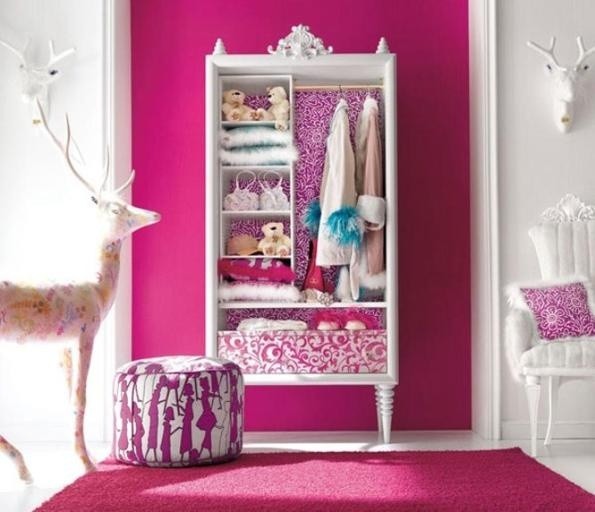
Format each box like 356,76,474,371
507,192,595,459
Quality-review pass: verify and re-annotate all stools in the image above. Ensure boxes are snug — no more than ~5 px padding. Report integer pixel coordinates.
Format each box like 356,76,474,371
115,356,245,467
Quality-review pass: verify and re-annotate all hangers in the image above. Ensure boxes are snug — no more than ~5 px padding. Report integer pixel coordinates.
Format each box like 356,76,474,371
363,85,374,112
334,83,347,107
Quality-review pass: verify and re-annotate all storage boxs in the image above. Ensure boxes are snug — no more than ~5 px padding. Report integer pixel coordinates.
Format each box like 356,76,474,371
216,329,387,373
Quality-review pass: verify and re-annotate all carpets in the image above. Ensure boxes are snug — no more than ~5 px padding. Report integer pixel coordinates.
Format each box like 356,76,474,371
36,447,595,511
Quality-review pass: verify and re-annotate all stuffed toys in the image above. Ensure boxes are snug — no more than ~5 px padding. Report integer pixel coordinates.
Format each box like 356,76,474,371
221,86,256,122
256,84,290,121
257,222,293,258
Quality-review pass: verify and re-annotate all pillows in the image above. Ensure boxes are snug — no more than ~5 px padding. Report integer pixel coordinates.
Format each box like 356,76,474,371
218,257,298,283
505,277,595,347
221,127,293,148
218,281,302,303
218,147,299,167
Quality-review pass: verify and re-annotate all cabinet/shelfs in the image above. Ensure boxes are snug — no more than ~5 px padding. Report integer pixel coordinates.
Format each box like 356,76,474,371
202,23,402,444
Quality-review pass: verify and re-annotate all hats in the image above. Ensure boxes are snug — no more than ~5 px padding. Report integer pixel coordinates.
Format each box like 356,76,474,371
227,234,264,256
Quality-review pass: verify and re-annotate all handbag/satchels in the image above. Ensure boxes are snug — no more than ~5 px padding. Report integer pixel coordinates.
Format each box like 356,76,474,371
223,170,259,210
257,170,289,211
